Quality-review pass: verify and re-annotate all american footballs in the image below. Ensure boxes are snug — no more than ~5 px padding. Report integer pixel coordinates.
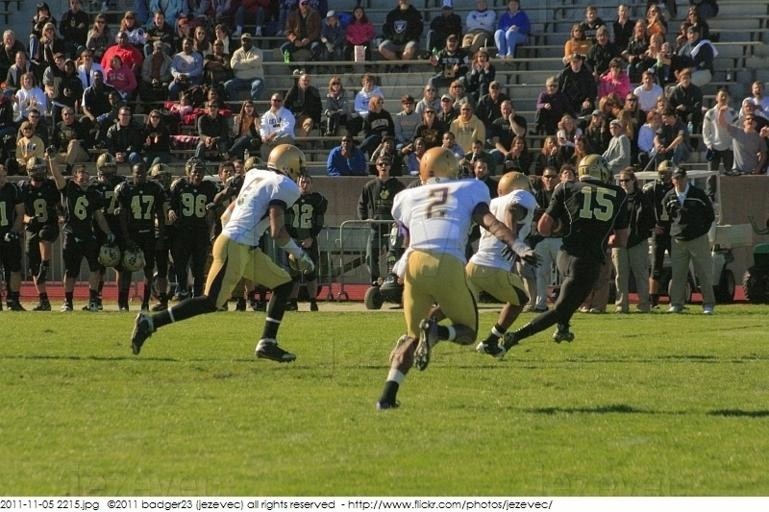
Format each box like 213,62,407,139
551,218,571,236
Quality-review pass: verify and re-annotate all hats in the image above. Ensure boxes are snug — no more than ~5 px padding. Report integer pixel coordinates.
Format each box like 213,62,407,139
672,167,685,178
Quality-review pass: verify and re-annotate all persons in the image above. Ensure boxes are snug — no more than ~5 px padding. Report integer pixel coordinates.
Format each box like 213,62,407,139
0,0,769,313
130,144,314,363
428,169,540,358
376,146,544,410
501,153,631,356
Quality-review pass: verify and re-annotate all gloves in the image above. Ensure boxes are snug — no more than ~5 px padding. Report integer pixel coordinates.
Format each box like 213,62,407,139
298,251,314,274
501,245,517,262
520,250,544,268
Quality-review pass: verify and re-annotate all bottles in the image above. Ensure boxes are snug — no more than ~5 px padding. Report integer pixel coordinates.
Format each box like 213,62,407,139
432,45,438,58
686,121,694,136
244,149,250,164
283,48,290,63
725,65,732,81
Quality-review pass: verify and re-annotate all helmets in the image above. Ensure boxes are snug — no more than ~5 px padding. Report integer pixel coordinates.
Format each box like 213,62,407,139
122,248,144,272
497,172,529,197
98,244,121,267
419,146,458,184
26,152,265,189
578,154,608,184
267,144,306,181
658,160,675,186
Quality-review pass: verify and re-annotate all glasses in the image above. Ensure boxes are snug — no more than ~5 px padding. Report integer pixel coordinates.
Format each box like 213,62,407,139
620,179,632,181
544,176,557,178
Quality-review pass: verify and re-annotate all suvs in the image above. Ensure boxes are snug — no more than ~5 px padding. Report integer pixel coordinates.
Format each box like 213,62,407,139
603,244,736,304
363,233,403,309
741,217,769,304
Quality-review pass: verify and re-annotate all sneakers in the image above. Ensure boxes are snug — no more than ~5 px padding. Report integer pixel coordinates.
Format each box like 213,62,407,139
131,312,155,355
377,317,439,408
254,339,295,361
476,304,714,360
